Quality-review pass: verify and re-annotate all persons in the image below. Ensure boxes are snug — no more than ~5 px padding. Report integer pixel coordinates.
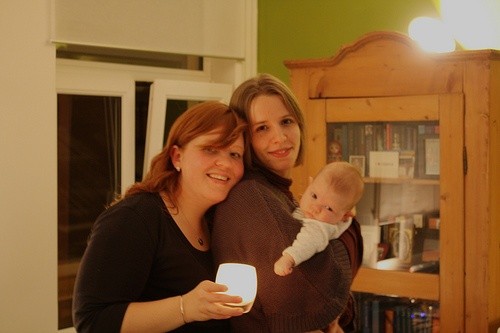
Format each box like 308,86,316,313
275,161,363,276
212,72,364,333
72,101,248,333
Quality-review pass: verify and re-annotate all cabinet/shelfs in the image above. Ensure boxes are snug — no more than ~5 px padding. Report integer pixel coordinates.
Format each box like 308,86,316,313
285,30,500,333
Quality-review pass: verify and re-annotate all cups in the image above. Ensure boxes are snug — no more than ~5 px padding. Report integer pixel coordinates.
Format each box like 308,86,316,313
213,263,257,313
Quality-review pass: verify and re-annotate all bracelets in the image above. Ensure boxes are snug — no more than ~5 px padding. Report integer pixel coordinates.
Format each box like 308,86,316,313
180,296,187,324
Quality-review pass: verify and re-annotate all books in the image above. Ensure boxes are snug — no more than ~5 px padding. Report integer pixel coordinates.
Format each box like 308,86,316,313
353,292,440,333
326,120,441,179
360,209,440,275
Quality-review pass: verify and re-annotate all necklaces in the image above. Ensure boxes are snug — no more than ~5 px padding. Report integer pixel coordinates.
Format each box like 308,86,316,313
174,193,205,245
